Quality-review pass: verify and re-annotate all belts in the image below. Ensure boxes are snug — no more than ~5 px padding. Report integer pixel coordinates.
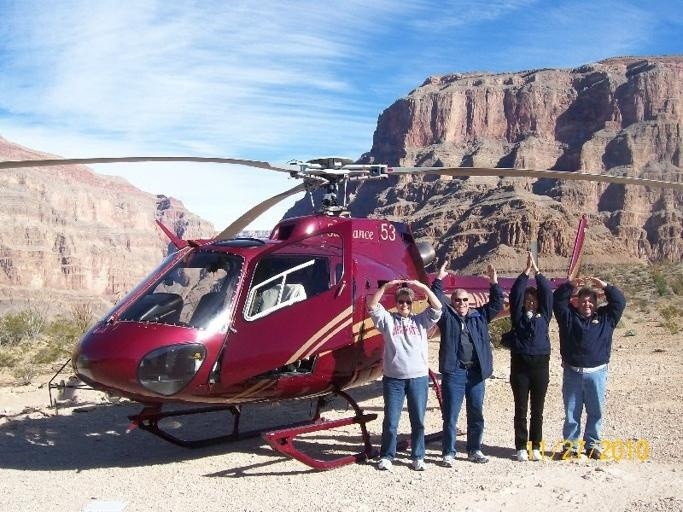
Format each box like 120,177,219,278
568,363,607,374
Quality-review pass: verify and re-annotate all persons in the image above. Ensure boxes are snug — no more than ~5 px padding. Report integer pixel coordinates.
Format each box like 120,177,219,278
551,276,627,461
429,258,503,467
506,249,553,462
366,278,442,471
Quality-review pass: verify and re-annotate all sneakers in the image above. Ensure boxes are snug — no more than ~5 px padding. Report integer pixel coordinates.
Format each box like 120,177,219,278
517,450,528,461
586,448,602,458
529,449,542,460
378,458,392,470
563,449,580,459
411,459,427,471
468,451,489,463
442,456,455,467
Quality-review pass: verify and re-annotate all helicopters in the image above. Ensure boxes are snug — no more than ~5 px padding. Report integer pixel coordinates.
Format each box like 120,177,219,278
0,151,682,473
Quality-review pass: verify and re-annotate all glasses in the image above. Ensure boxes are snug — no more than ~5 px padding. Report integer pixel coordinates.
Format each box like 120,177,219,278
454,298,469,302
524,297,538,304
398,299,412,305
